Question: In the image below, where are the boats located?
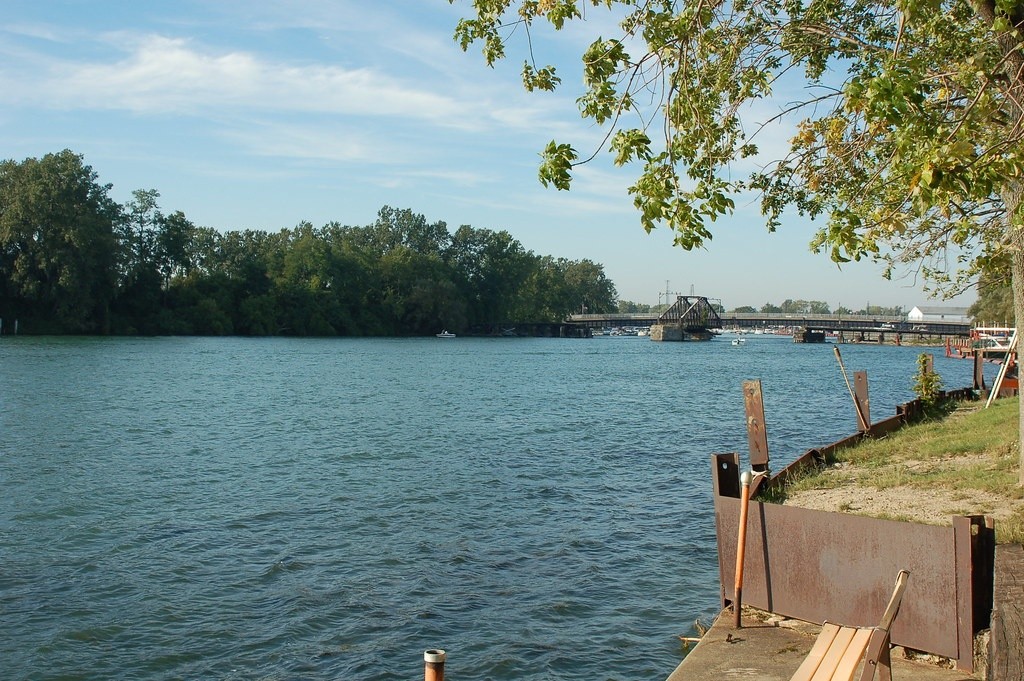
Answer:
[591,324,651,337]
[436,330,456,338]
[731,339,746,347]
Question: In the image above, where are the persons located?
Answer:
[1005,361,1019,380]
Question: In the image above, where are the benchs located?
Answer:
[789,570,910,681]
